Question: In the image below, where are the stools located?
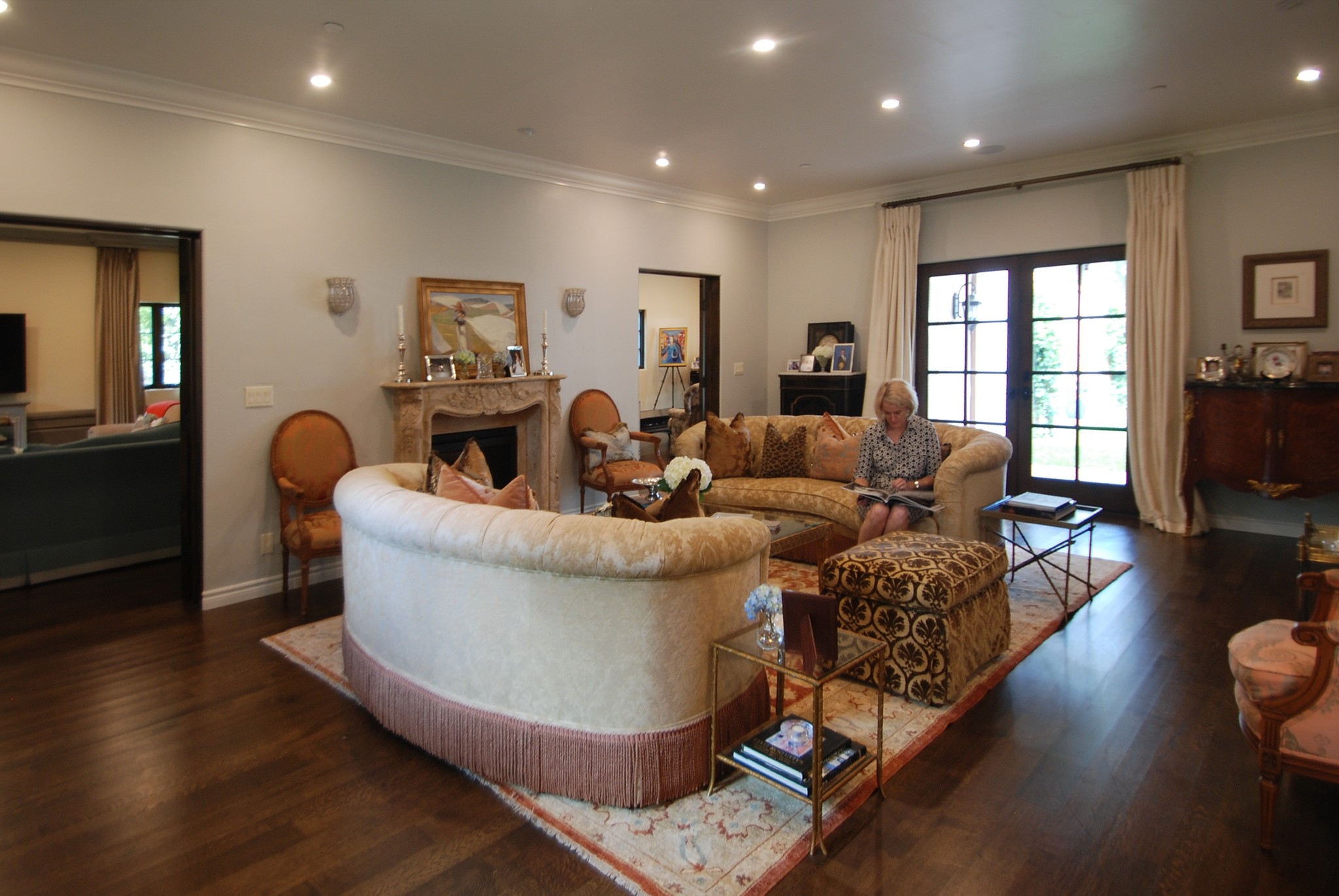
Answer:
[818,529,1012,709]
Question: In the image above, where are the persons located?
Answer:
[514,351,524,374]
[437,363,444,372]
[853,379,941,545]
[793,362,798,370]
[838,349,847,370]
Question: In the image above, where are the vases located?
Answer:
[494,362,507,377]
[457,363,470,380]
[755,609,783,652]
[816,354,829,373]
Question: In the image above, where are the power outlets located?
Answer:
[261,532,275,556]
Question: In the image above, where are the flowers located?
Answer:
[654,455,713,503]
[744,582,795,645]
[811,344,834,368]
[451,350,476,365]
[490,350,513,368]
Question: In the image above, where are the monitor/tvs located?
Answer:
[0,313,26,393]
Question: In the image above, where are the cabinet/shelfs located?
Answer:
[689,368,700,387]
[0,392,32,448]
[776,369,867,418]
[1177,375,1339,538]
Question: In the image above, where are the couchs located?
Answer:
[667,410,1015,566]
[331,436,773,813]
[0,399,183,592]
[666,382,701,455]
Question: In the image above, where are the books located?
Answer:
[733,714,867,797]
[839,482,945,512]
[999,491,1076,521]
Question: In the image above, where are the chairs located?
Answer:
[1225,565,1339,858]
[270,409,361,617]
[568,387,666,516]
[1294,509,1339,621]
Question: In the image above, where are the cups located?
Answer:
[477,355,494,378]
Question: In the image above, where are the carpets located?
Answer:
[259,538,1136,896]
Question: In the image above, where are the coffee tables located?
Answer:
[596,492,833,595]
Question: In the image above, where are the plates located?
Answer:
[1259,343,1298,380]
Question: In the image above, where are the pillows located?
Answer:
[820,411,852,440]
[754,422,811,479]
[434,463,541,512]
[581,423,638,476]
[422,437,495,496]
[610,467,705,525]
[702,412,756,481]
[806,422,864,483]
[132,414,167,432]
[940,441,953,463]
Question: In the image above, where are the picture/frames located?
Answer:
[424,354,457,381]
[1306,354,1339,382]
[780,588,839,672]
[787,358,801,372]
[658,327,688,367]
[417,276,530,382]
[1241,248,1331,331]
[1250,340,1310,380]
[830,342,856,373]
[505,345,528,378]
[798,353,816,373]
[1200,354,1225,381]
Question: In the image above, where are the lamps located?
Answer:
[953,281,983,334]
[566,288,587,317]
[326,277,355,314]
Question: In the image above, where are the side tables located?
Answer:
[977,493,1104,625]
[703,609,888,859]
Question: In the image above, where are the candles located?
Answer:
[396,305,405,335]
[542,308,547,334]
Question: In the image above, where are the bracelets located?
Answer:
[914,480,919,489]
